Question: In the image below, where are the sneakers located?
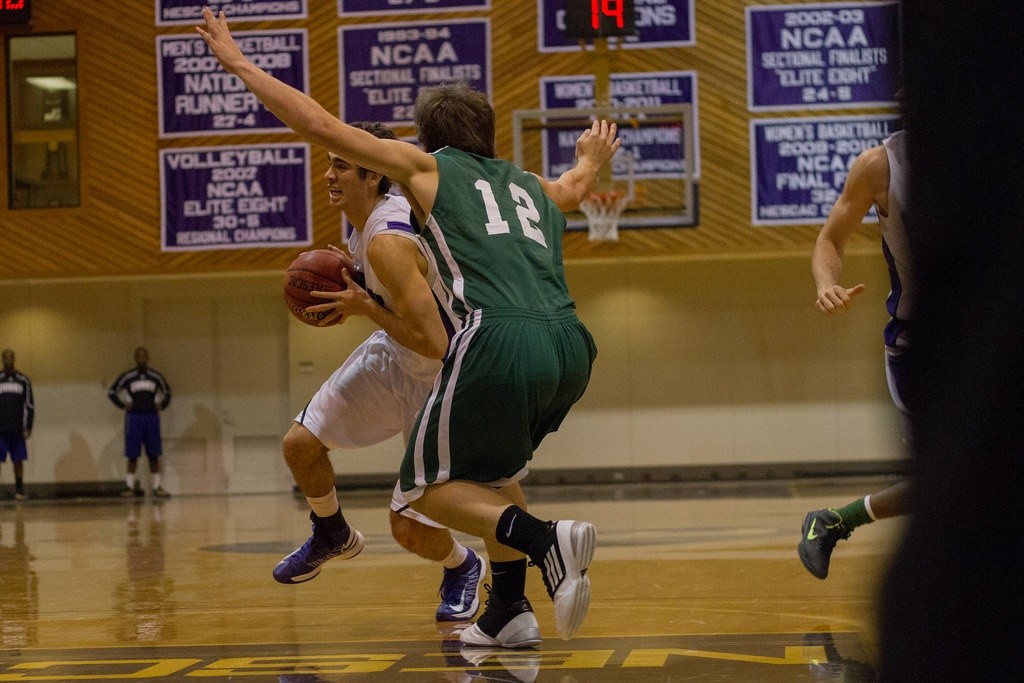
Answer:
[528,520,597,641]
[435,547,487,622]
[460,583,542,648]
[271,524,365,585]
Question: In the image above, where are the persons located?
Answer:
[107,346,172,500]
[193,6,622,649]
[798,81,914,581]
[0,348,35,502]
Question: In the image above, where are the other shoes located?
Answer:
[798,508,852,579]
[153,486,172,499]
[14,493,30,500]
[118,487,137,499]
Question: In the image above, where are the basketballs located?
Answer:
[283,248,357,328]
